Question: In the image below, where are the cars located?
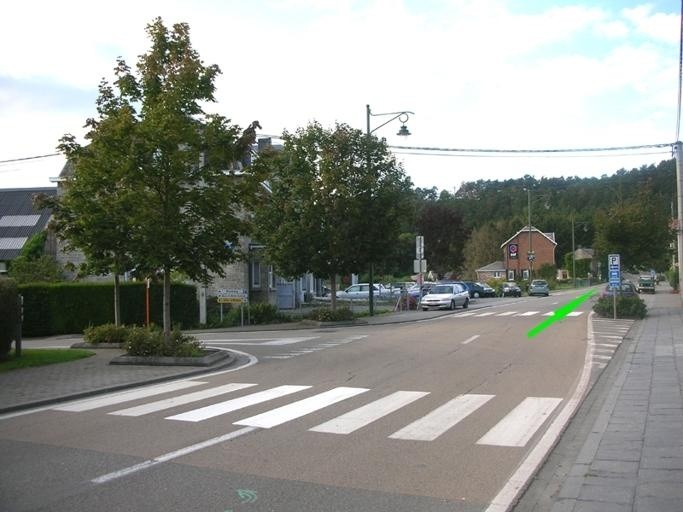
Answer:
[502,282,522,298]
[638,275,656,294]
[602,280,638,298]
[326,280,496,311]
[528,279,549,297]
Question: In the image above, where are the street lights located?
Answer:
[366,104,415,317]
